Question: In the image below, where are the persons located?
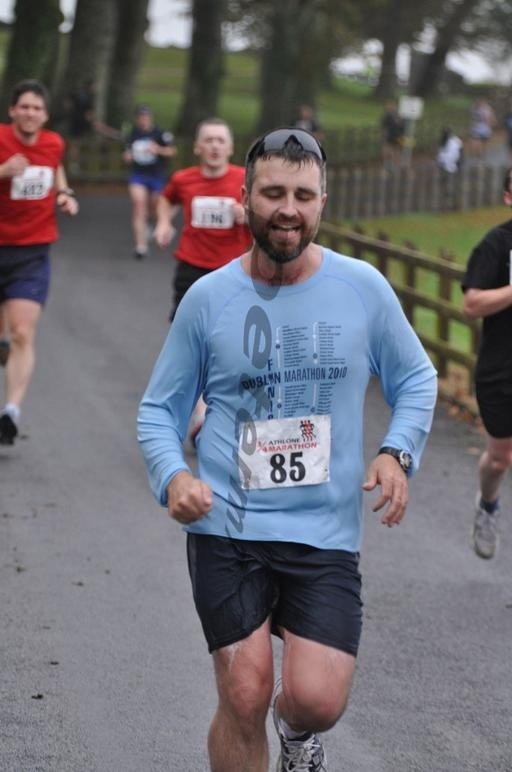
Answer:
[139,126,442,772]
[436,122,465,175]
[294,100,326,144]
[457,160,512,563]
[380,97,409,170]
[151,114,255,451]
[469,94,497,153]
[116,104,176,261]
[0,76,80,447]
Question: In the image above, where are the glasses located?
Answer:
[247,130,324,166]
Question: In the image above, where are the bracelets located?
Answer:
[55,186,75,195]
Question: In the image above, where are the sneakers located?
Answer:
[134,249,146,258]
[2,415,13,448]
[474,493,499,556]
[271,677,325,771]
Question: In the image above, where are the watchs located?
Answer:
[375,442,417,481]
